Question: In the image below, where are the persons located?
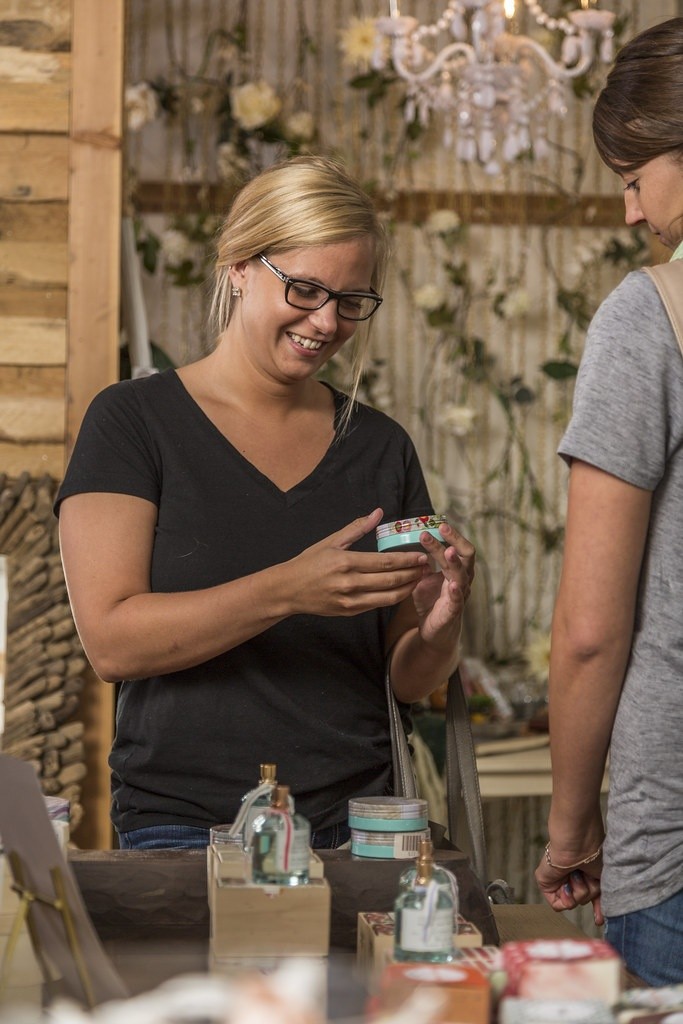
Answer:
[54,156,474,849]
[535,18,683,987]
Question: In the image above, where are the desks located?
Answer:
[70,850,502,955]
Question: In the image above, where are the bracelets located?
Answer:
[545,841,603,870]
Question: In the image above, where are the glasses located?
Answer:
[256,253,383,321]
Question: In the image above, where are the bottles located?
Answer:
[395,835,460,963]
[242,761,312,884]
